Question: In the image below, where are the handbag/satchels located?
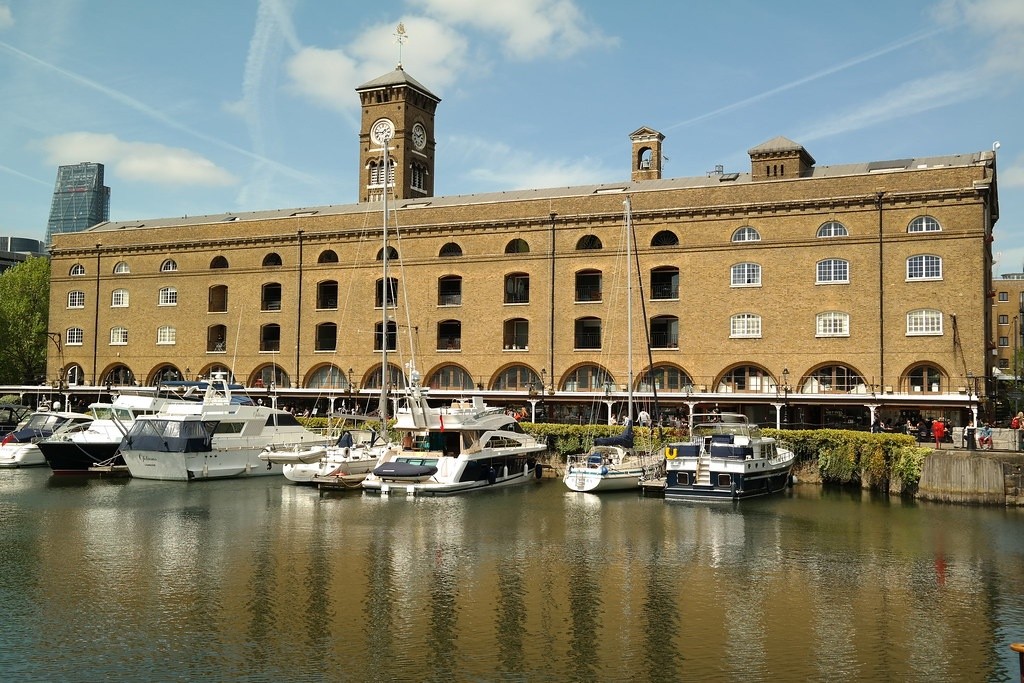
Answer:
[515,414,521,420]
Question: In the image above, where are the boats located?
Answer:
[0,304,340,483]
[664,412,795,504]
[257,446,327,465]
[361,360,547,497]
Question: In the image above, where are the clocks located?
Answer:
[371,118,395,145]
[412,123,426,151]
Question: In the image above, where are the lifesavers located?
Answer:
[666,448,677,459]
[343,447,350,457]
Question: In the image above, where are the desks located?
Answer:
[296,414,304,416]
[908,429,919,436]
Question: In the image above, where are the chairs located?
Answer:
[980,433,993,449]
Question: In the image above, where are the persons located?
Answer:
[978,422,992,450]
[964,421,973,440]
[895,418,903,426]
[885,418,893,425]
[508,407,527,422]
[873,419,886,433]
[623,415,628,426]
[636,408,650,426]
[933,417,952,448]
[291,403,296,415]
[658,415,663,425]
[1014,412,1024,429]
[918,421,926,432]
[303,407,311,417]
[610,414,615,425]
[401,432,414,450]
[283,403,289,411]
[904,420,912,429]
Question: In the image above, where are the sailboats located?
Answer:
[256,135,397,487]
[562,196,667,492]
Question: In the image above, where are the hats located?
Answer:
[984,423,990,425]
